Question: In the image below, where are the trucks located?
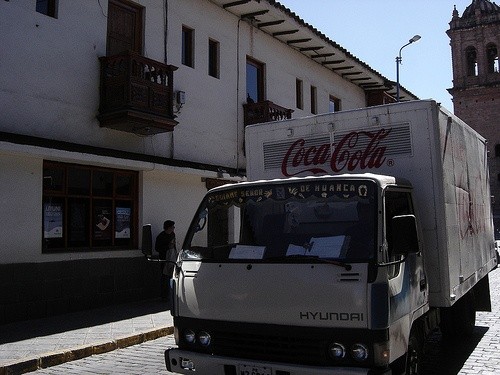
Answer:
[140,96,499,375]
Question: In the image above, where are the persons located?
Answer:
[348,202,372,256]
[154,220,177,311]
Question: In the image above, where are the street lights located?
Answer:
[395,34,422,102]
